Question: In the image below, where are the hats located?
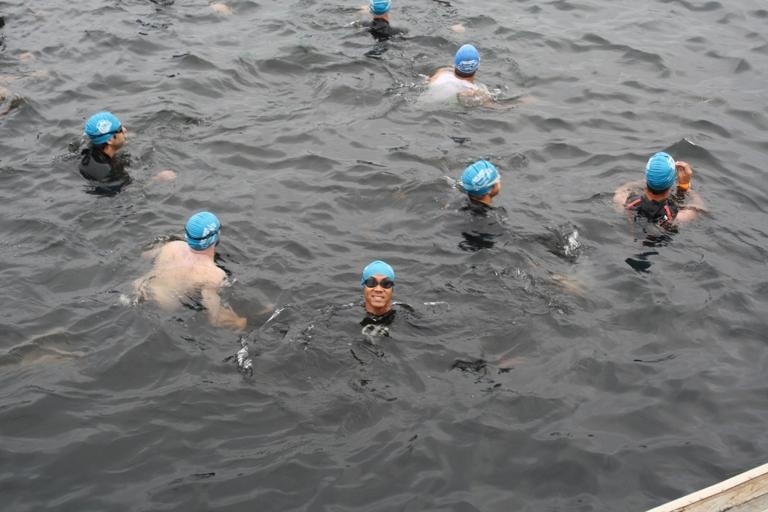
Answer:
[454,44,480,74]
[183,212,220,251]
[460,160,501,196]
[84,112,122,145]
[360,260,395,290]
[644,152,676,190]
[369,0,392,15]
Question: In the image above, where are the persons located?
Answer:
[413,43,538,112]
[132,209,277,331]
[81,110,177,197]
[337,258,416,340]
[349,1,467,45]
[608,147,710,229]
[450,156,508,239]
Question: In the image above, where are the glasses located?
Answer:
[365,277,394,289]
[109,125,122,135]
